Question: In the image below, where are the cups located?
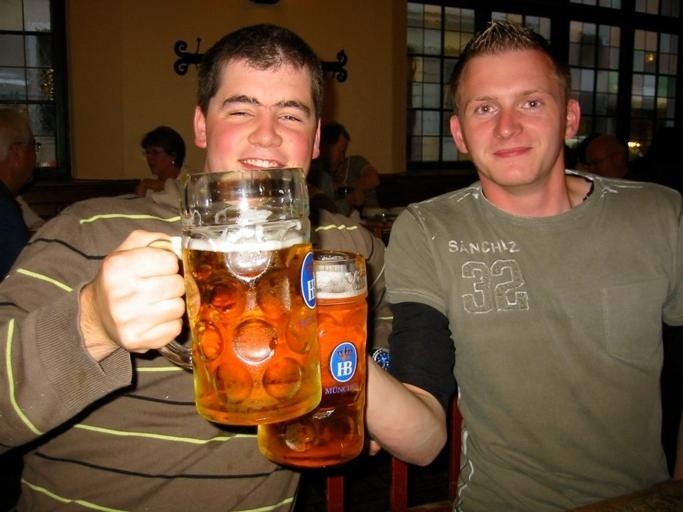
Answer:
[140,164,324,427]
[255,248,372,472]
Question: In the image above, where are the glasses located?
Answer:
[7,141,42,152]
[142,151,164,159]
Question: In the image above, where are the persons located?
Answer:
[305,121,349,226]
[0,22,395,509]
[136,124,198,195]
[580,132,633,180]
[364,12,681,512]
[0,102,60,279]
[333,151,382,217]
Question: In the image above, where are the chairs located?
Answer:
[327,391,462,512]
